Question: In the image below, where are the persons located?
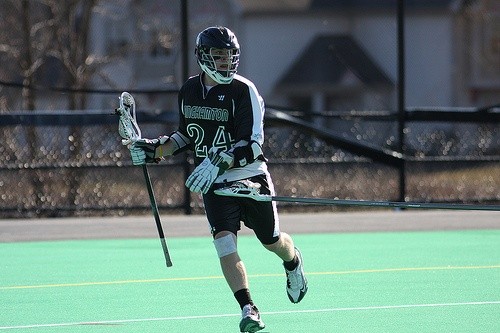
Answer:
[122,27,309,333]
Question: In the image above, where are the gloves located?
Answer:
[185,147,234,194]
[130,139,162,165]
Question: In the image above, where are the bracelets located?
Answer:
[159,146,162,158]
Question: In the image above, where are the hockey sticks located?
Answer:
[213,179,500,211]
[117,91,172,268]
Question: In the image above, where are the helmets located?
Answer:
[195,26,240,84]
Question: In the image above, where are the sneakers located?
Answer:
[240,304,265,332]
[283,246,308,303]
